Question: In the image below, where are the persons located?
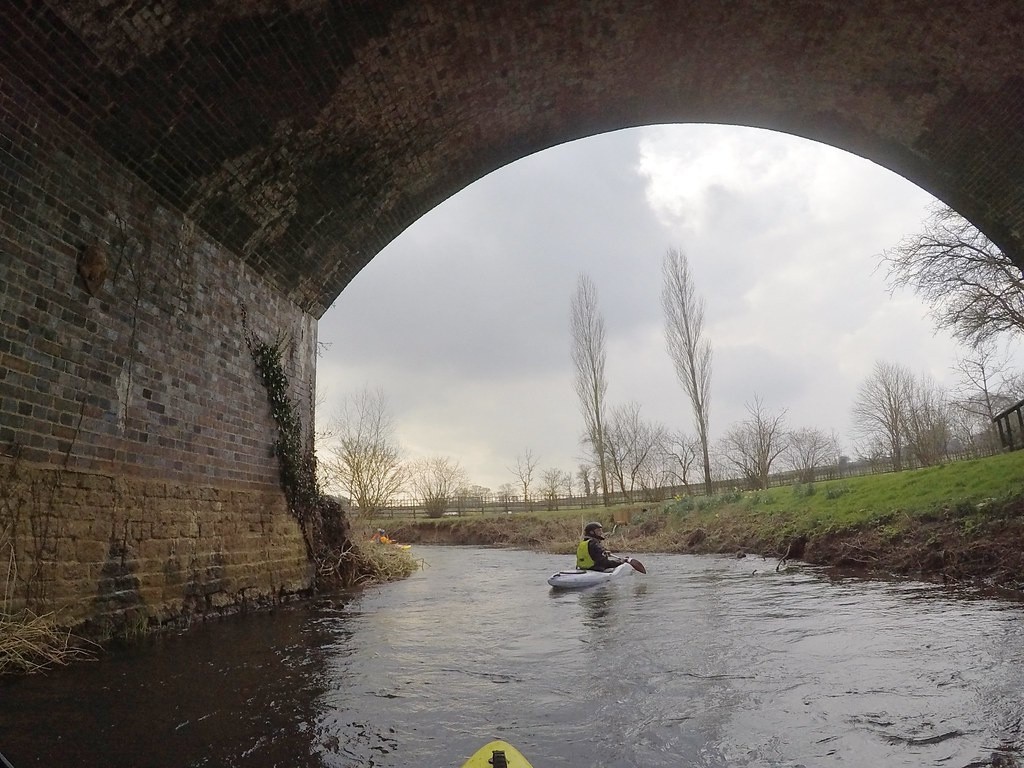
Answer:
[576,522,629,572]
[372,528,388,540]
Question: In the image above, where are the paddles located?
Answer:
[610,553,646,574]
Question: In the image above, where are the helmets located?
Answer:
[584,522,602,531]
[380,529,385,535]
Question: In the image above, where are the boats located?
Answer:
[369,540,412,550]
[547,555,635,589]
